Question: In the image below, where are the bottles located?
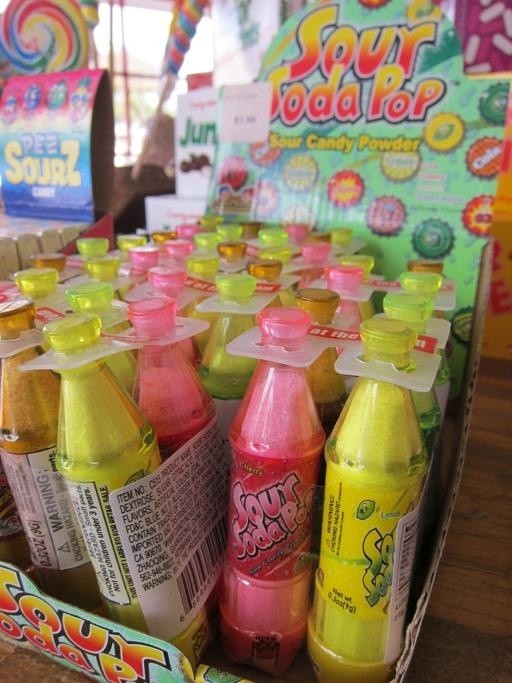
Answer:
[1,216,458,683]
